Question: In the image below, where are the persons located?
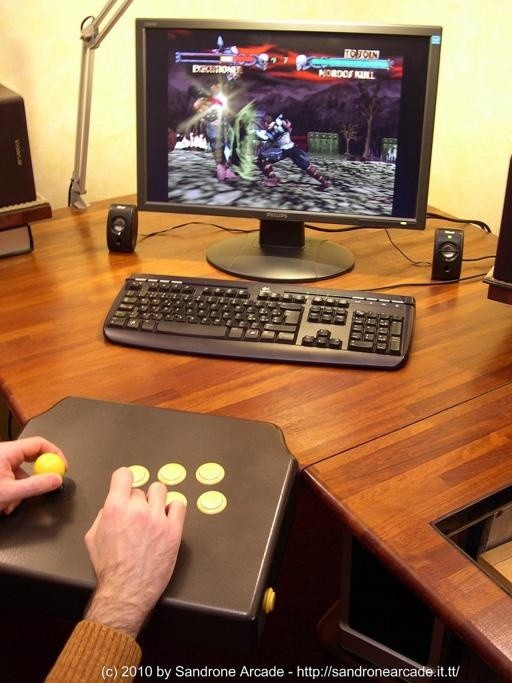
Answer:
[193,85,238,177]
[248,112,332,192]
[1,437,186,682]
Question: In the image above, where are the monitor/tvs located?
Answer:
[136,18,442,230]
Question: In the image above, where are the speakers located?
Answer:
[432,230,465,282]
[107,204,137,252]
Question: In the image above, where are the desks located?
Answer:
[0,191,512,683]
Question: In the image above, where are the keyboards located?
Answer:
[102,271,416,371]
